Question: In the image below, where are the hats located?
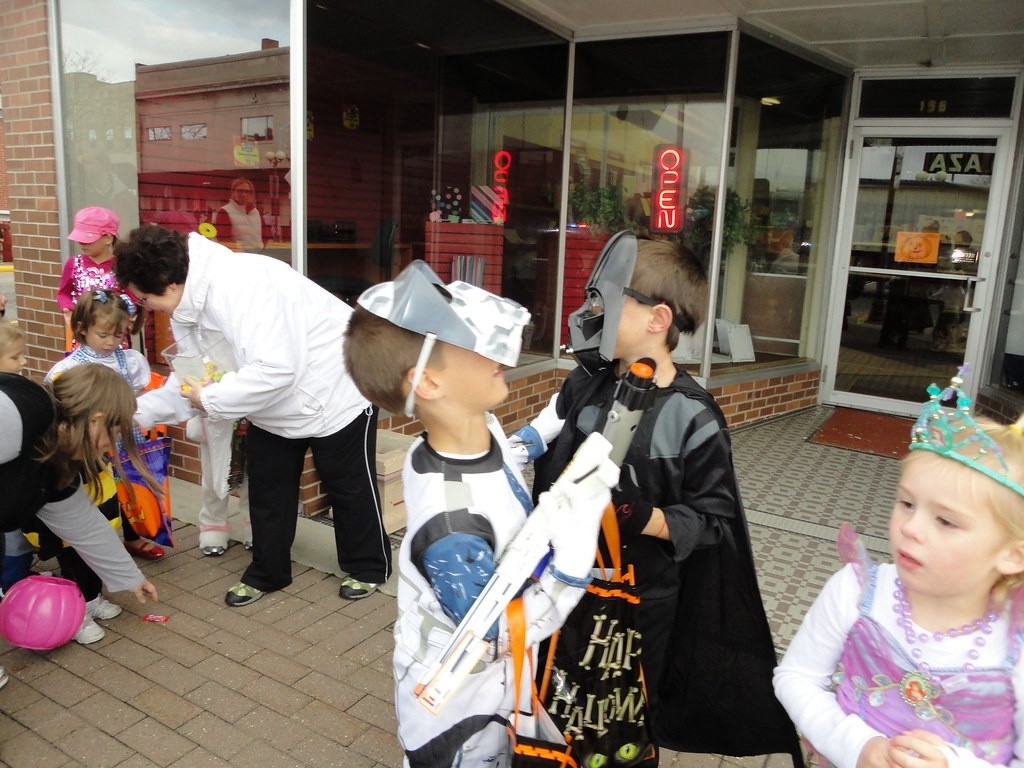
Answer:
[67,206,120,244]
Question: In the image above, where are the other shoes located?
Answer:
[924,337,949,353]
[0,571,58,604]
[876,330,909,351]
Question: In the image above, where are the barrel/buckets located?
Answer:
[161,329,239,392]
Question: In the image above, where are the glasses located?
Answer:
[233,188,252,194]
[133,292,149,308]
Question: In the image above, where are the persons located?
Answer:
[113,224,393,607]
[841,219,973,356]
[773,361,1024,767]
[0,322,41,597]
[44,289,166,559]
[526,229,802,768]
[0,364,159,688]
[215,177,265,253]
[44,448,125,644]
[56,206,145,352]
[189,410,254,556]
[343,258,615,768]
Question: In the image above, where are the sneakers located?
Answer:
[71,613,105,646]
[85,592,122,620]
[244,541,253,550]
[338,575,380,599]
[202,545,224,556]
[225,582,268,606]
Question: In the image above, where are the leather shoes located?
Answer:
[122,540,164,561]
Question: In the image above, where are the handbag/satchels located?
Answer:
[105,424,174,548]
[506,596,583,768]
[532,498,660,768]
